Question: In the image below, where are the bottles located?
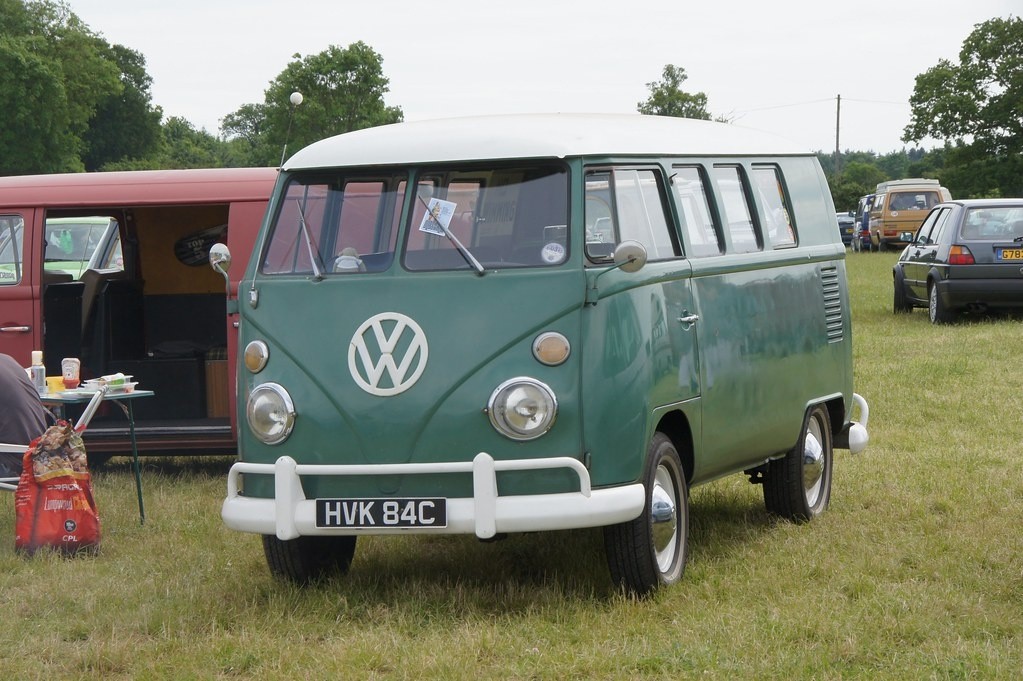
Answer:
[30,350,46,397]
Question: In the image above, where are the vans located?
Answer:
[864,178,954,252]
[849,194,876,252]
[0,171,484,473]
[207,112,870,603]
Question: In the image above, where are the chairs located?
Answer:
[42,273,86,368]
[77,267,128,346]
[964,225,979,238]
[1011,220,1023,238]
[0,384,109,554]
[914,201,925,210]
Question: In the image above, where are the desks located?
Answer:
[40,390,155,526]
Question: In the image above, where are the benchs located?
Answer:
[204,339,230,418]
[509,243,614,265]
[105,280,204,420]
[320,245,499,273]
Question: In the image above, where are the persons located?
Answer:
[0,353,48,486]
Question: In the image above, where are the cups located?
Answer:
[47,377,65,394]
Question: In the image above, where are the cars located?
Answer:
[836,211,857,245]
[892,198,1023,326]
